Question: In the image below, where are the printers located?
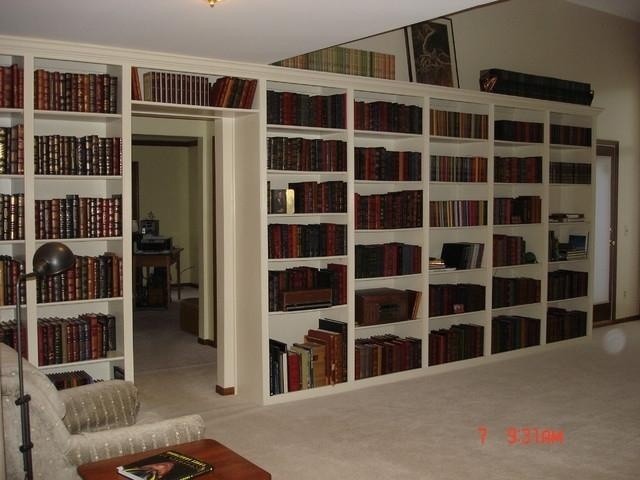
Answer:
[140,235,173,252]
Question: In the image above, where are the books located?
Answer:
[117,450,212,480]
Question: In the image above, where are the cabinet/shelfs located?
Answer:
[427,92,491,368]
[546,107,591,350]
[127,61,261,115]
[491,101,544,363]
[349,79,426,389]
[0,51,134,390]
[236,71,352,408]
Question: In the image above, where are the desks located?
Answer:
[78,436,271,479]
[138,248,183,309]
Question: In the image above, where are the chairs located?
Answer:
[0,335,207,480]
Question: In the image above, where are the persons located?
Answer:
[0,58,589,396]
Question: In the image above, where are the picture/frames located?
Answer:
[405,18,461,87]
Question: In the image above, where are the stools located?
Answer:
[180,297,198,336]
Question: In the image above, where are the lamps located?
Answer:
[15,242,76,480]
